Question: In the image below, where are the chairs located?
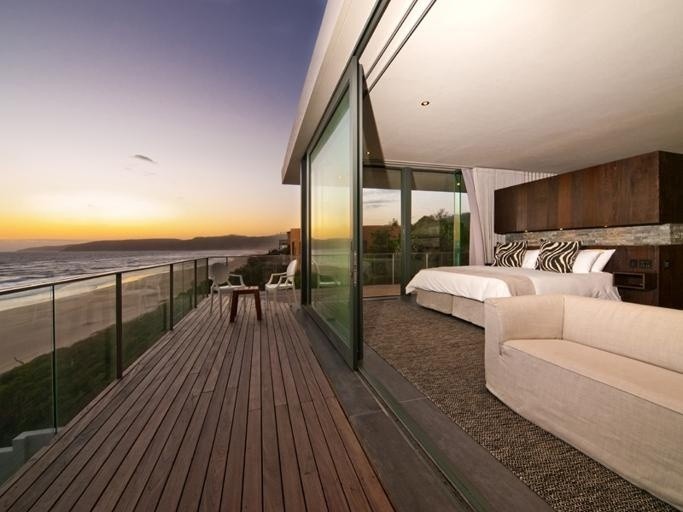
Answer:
[311,260,341,309]
[263,259,297,316]
[207,260,246,317]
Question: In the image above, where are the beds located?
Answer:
[404,245,623,330]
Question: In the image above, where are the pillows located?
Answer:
[521,250,536,267]
[584,248,616,272]
[572,250,604,273]
[492,240,528,268]
[532,239,581,273]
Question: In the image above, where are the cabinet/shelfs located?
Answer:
[526,174,560,231]
[494,176,527,233]
[606,151,659,228]
[560,162,606,228]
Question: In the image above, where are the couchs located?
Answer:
[483,292,682,509]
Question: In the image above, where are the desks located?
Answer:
[229,285,261,322]
